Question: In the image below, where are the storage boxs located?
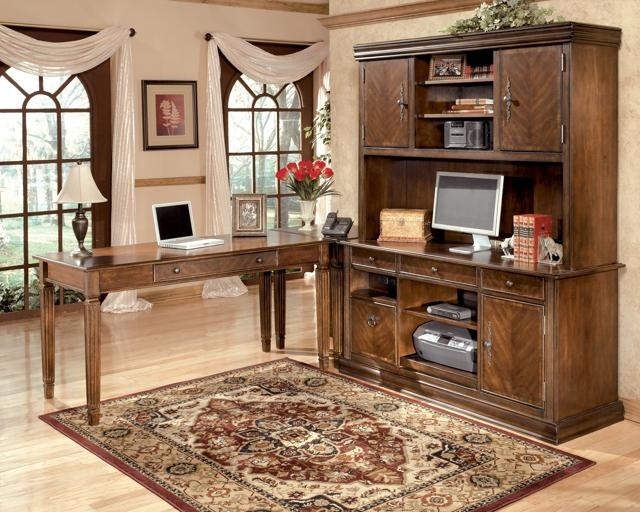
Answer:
[378,209,432,244]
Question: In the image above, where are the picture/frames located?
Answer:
[232,194,267,239]
[141,80,199,151]
[428,54,465,80]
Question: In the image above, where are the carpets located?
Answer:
[39,357,596,512]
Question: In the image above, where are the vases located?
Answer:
[297,200,318,231]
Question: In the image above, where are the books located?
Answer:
[444,97,497,114]
[465,65,494,79]
[513,213,554,266]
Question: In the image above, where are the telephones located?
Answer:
[321,210,355,238]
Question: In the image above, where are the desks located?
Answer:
[31,230,333,426]
[269,224,359,368]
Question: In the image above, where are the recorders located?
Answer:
[444,117,491,150]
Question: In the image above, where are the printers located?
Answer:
[413,321,478,374]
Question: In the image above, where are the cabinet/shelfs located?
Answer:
[332,21,624,445]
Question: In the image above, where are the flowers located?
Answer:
[276,161,341,201]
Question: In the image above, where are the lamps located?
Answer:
[52,161,107,259]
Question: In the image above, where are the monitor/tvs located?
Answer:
[432,170,503,254]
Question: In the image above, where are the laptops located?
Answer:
[152,200,225,250]
